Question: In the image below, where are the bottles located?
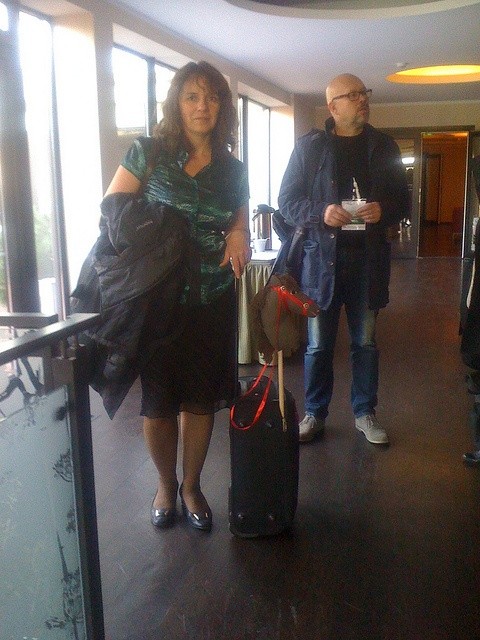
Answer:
[252,203,275,251]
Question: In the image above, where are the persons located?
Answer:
[277,73,409,443]
[102,60,252,529]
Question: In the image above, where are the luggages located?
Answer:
[229,349,300,540]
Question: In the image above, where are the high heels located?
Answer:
[151,479,179,527]
[179,483,215,531]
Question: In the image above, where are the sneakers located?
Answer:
[298,409,326,445]
[355,413,389,445]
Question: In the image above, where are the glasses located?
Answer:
[334,88,373,101]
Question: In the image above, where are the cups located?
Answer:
[253,239,267,253]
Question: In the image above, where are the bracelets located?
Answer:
[225,228,250,235]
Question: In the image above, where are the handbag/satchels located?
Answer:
[266,213,301,287]
